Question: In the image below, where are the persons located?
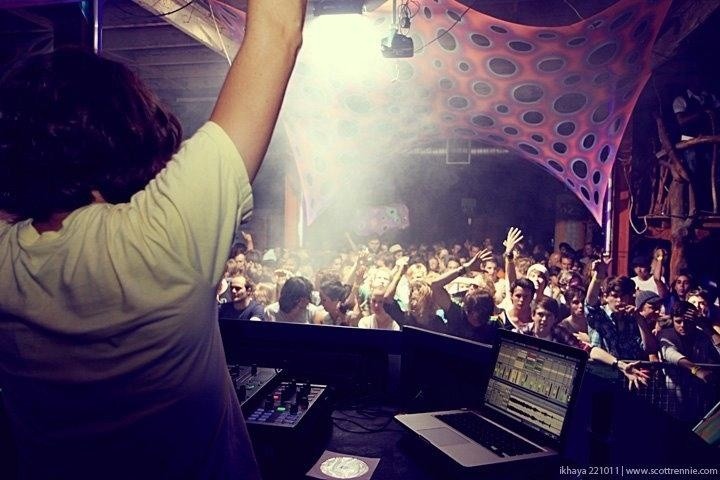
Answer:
[0,1,307,480]
[220,226,719,390]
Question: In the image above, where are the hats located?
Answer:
[389,244,402,253]
[526,263,549,278]
[632,256,650,266]
[634,291,662,309]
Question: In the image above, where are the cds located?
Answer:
[320,457,369,479]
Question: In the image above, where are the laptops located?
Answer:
[391,328,588,480]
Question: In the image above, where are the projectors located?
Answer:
[380,36,414,57]
